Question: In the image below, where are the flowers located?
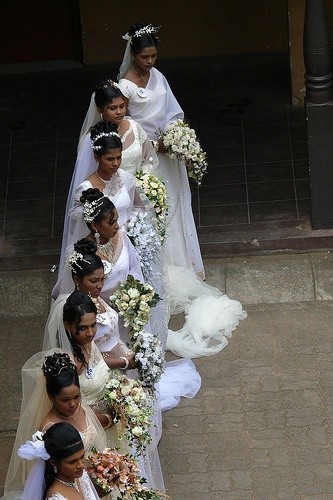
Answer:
[87,117,208,500]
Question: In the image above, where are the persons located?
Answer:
[41,290,118,498]
[17,420,101,500]
[39,352,123,500]
[116,19,205,282]
[61,79,168,413]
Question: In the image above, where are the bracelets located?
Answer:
[102,414,112,429]
[151,140,159,153]
[118,356,130,371]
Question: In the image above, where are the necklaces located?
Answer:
[95,169,110,184]
[51,405,81,423]
[77,343,92,357]
[87,234,116,263]
[54,477,80,493]
[116,120,121,131]
[132,64,148,84]
